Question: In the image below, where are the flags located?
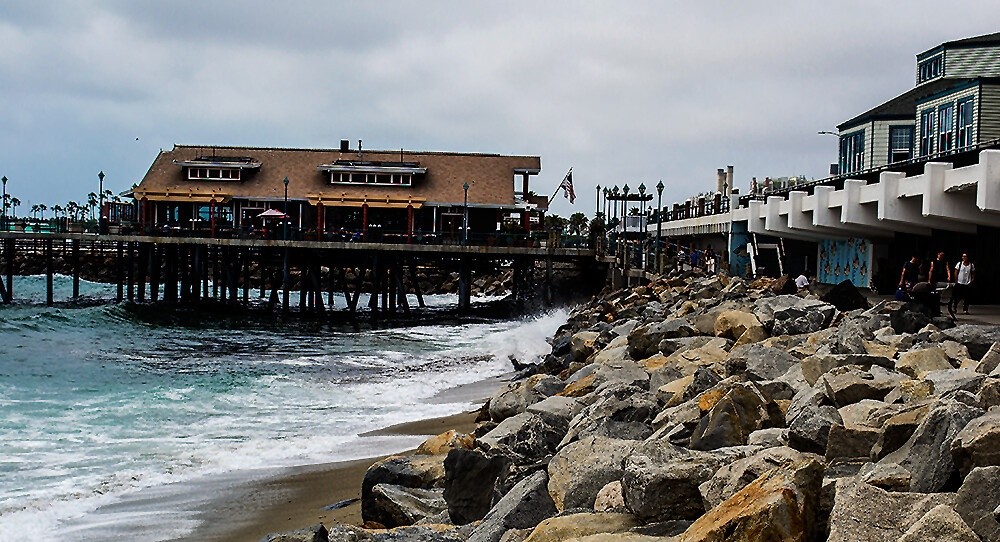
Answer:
[562,169,576,204]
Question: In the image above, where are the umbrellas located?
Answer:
[256,208,289,219]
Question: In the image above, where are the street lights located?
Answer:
[602,187,607,225]
[98,171,105,235]
[656,180,664,255]
[594,184,601,217]
[621,184,629,247]
[606,188,612,225]
[2,176,8,223]
[613,184,619,220]
[637,183,647,254]
[462,181,469,249]
[282,177,289,240]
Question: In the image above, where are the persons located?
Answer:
[952,252,977,314]
[794,270,810,289]
[898,253,921,297]
[645,233,717,274]
[927,249,951,284]
